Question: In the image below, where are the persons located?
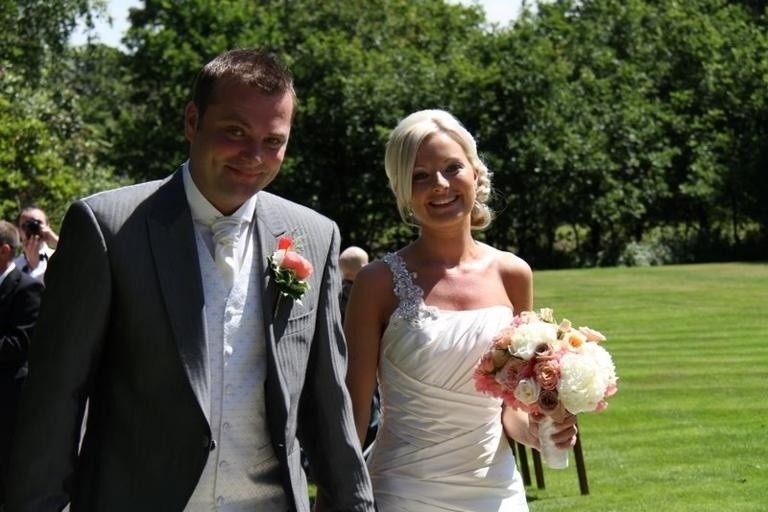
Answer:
[1,49,374,512]
[14,207,59,279]
[342,108,578,512]
[340,245,389,330]
[0,221,46,392]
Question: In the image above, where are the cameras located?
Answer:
[18,219,43,240]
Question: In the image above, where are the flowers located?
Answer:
[471,307,620,426]
[267,226,316,307]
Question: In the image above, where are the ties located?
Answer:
[210,218,241,289]
